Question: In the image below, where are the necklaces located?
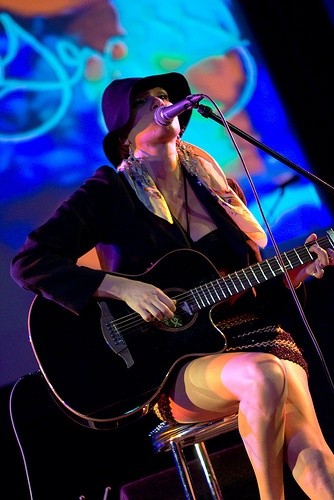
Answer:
[158,167,191,242]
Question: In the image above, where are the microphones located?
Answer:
[153,93,206,127]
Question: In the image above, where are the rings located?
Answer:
[312,271,316,277]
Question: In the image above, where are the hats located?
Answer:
[101,72,192,169]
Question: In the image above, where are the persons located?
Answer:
[8,73,334,500]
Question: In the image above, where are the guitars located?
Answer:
[27,226,334,421]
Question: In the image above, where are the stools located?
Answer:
[148,409,241,499]
